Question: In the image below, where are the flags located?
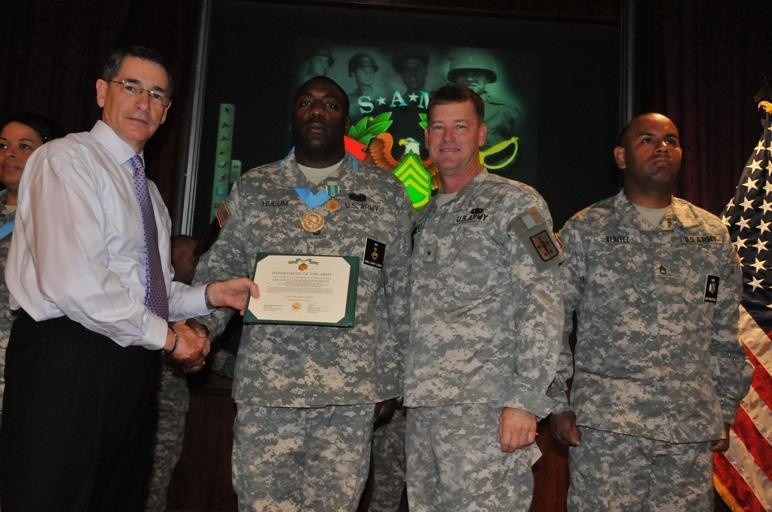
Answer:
[709,115,771,512]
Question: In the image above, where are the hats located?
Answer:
[392,45,429,74]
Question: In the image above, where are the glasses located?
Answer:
[105,79,171,108]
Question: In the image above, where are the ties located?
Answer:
[130,155,168,322]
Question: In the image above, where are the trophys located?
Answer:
[301,211,325,232]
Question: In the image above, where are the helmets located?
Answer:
[446,47,498,83]
[349,53,377,77]
[313,46,333,66]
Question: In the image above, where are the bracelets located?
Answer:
[166,323,178,355]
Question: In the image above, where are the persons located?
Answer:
[1,117,47,407]
[145,234,236,511]
[301,34,528,174]
[548,112,755,511]
[400,84,566,511]
[370,399,412,511]
[2,40,260,510]
[175,77,414,511]
